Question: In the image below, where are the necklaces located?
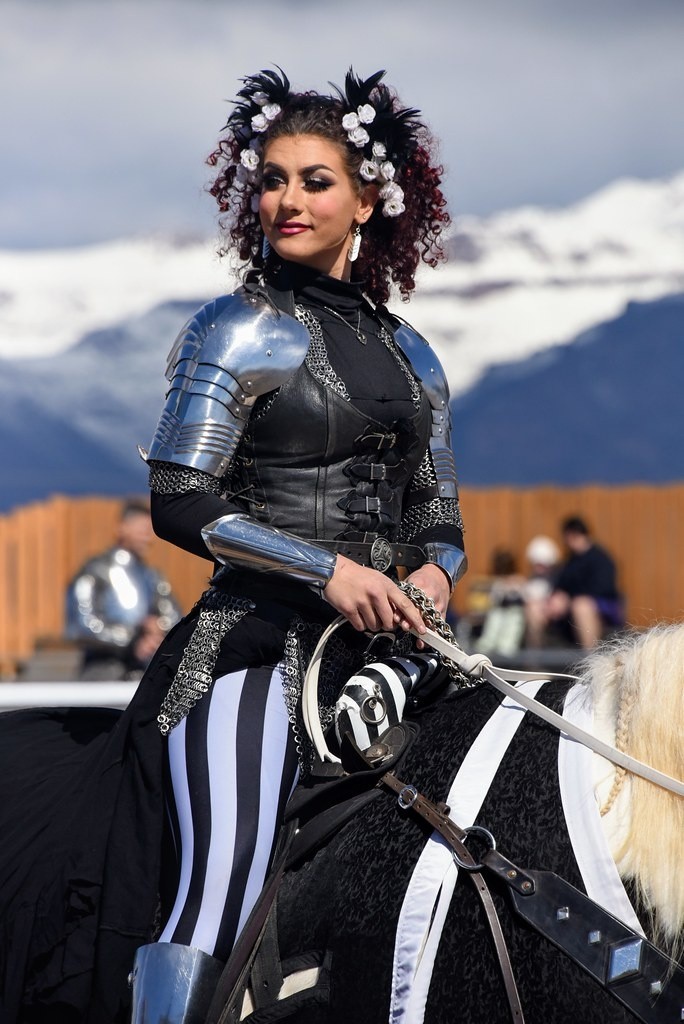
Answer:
[321,306,367,346]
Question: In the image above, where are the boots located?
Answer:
[127,942,225,1023]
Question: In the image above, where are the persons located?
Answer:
[0,64,469,1024]
[465,517,627,657]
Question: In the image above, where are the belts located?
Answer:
[301,538,429,573]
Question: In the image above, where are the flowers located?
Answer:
[379,182,404,202]
[348,126,370,148]
[249,91,269,106]
[251,113,270,132]
[371,140,387,164]
[239,148,260,171]
[262,103,281,121]
[377,161,395,184]
[342,112,360,132]
[250,193,260,212]
[381,199,406,218]
[357,104,376,124]
[359,160,381,181]
[236,163,253,185]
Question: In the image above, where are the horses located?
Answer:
[0,625,684,1024]
